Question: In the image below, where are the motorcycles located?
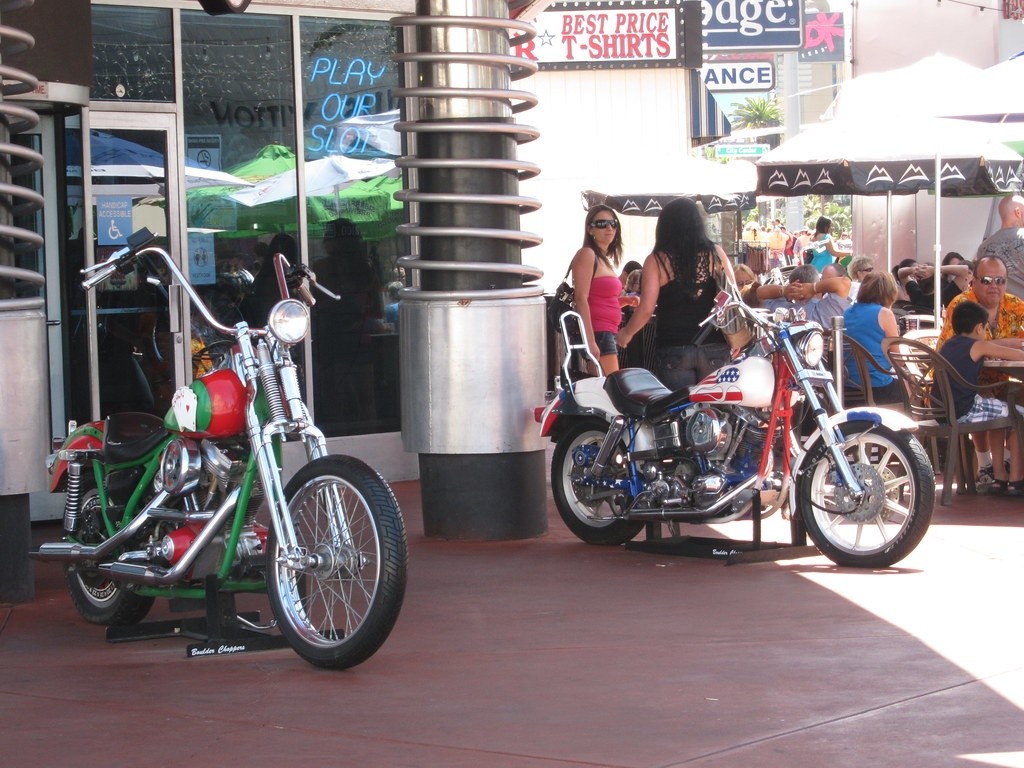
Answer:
[26,226,410,670]
[535,290,936,569]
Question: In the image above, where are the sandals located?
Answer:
[1004,481,1024,496]
[990,479,1008,495]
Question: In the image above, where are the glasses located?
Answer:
[736,280,753,288]
[856,266,873,273]
[588,218,618,229]
[973,273,1008,285]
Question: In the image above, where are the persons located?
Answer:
[619,217,974,473]
[181,218,406,381]
[931,255,1024,494]
[573,205,640,377]
[619,198,753,395]
[931,302,1024,494]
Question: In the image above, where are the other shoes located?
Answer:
[974,459,1010,496]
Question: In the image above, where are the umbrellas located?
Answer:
[64,109,404,281]
[580,55,1024,339]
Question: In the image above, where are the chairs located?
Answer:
[823,315,1024,507]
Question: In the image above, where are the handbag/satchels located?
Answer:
[710,242,752,349]
[547,245,598,337]
